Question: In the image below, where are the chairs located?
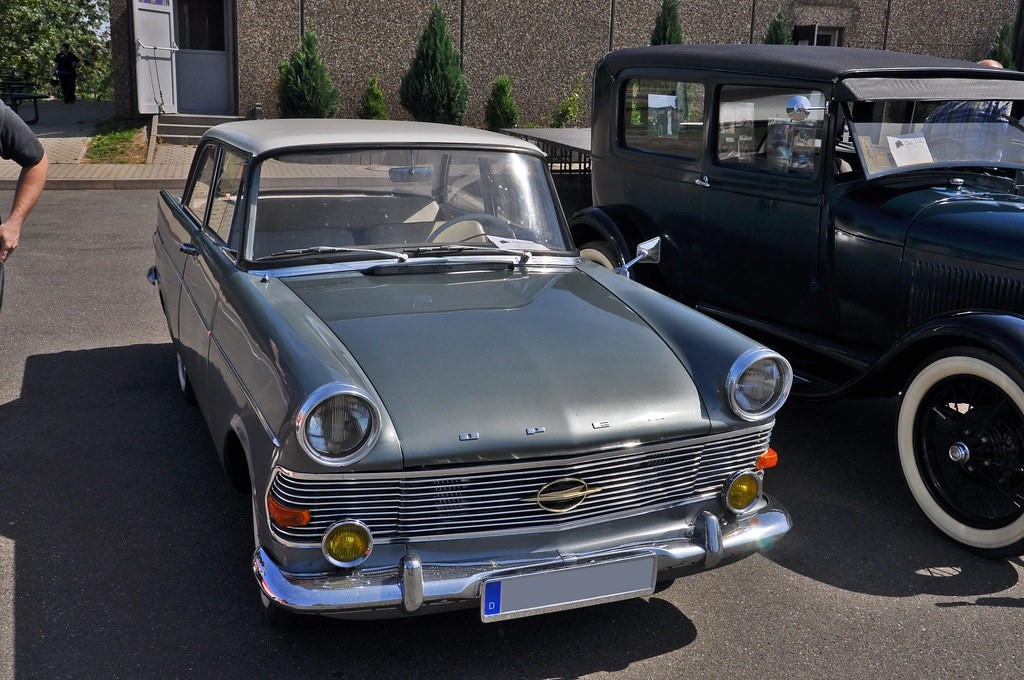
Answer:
[255,228,356,260]
[366,220,487,245]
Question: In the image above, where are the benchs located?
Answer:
[0,85,50,99]
[259,194,440,247]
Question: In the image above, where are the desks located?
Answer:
[0,80,39,124]
[501,128,591,210]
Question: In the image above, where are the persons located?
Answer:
[50,42,84,107]
[911,60,1024,162]
[0,99,48,317]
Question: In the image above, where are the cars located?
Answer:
[147,117,797,625]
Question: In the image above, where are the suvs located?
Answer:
[559,42,1024,558]
